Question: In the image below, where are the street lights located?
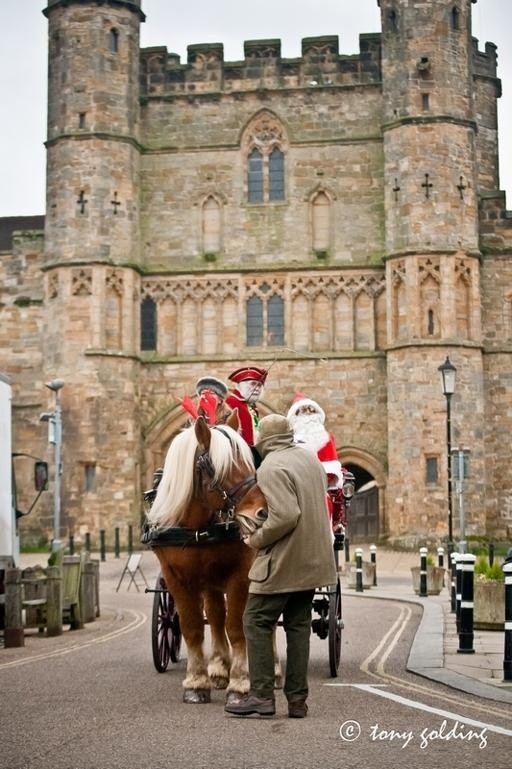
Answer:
[438,353,459,615]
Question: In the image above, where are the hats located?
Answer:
[228,366,268,384]
[195,375,227,401]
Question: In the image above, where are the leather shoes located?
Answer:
[224,696,276,716]
[287,702,309,718]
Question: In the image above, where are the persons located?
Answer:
[195,366,342,718]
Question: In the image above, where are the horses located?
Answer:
[142,407,270,708]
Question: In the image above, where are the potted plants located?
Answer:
[470,551,506,631]
[346,557,376,589]
[410,554,446,595]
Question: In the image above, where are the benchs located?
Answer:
[21,551,84,632]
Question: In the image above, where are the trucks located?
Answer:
[0,372,51,635]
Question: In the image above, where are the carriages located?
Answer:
[138,404,349,710]
